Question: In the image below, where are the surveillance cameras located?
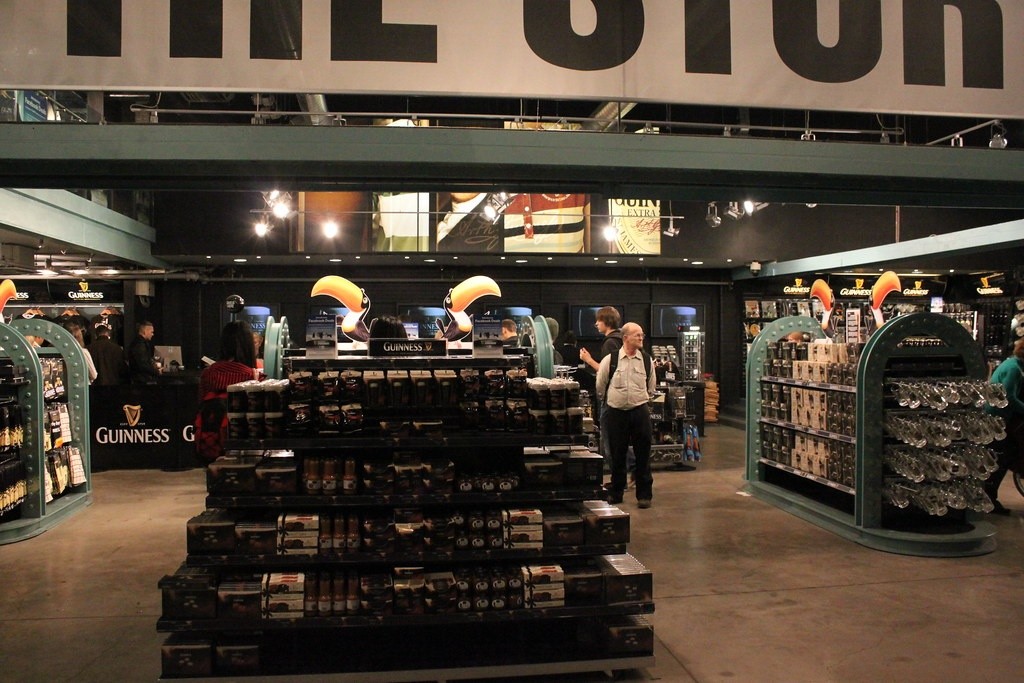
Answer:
[750,261,761,276]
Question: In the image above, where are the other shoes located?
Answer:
[603,490,625,506]
[636,497,652,510]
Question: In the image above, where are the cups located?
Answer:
[882,477,994,516]
[882,446,999,484]
[890,381,1008,411]
[882,413,1007,449]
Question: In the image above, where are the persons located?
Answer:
[788,332,803,343]
[982,337,1024,514]
[580,305,636,493]
[596,322,656,508]
[11,315,164,385]
[435,192,500,251]
[195,320,263,462]
[502,317,592,392]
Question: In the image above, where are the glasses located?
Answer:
[631,333,646,340]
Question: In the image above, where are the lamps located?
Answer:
[724,201,745,221]
[252,214,275,237]
[988,122,1008,149]
[745,200,770,216]
[663,218,680,238]
[704,201,722,229]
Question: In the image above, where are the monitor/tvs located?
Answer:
[219,302,281,338]
[569,304,625,341]
[397,303,452,339]
[307,302,350,319]
[484,303,541,323]
[154,346,183,370]
[651,303,706,337]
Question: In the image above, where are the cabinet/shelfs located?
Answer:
[600,386,685,469]
[0,318,92,542]
[154,353,656,683]
[680,331,701,382]
[739,295,1014,556]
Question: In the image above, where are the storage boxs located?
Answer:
[157,366,655,678]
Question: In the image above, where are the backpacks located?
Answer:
[192,384,235,465]
